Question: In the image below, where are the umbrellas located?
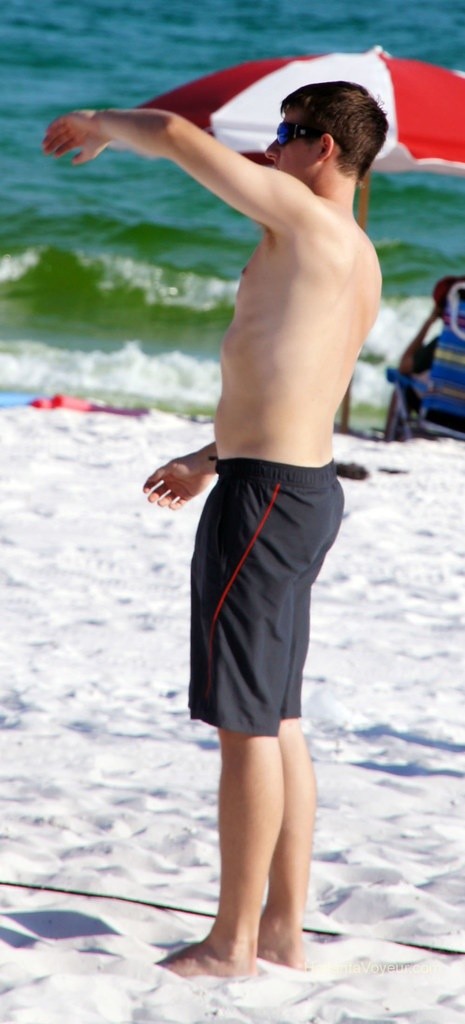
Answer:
[119,44,465,172]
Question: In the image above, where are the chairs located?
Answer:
[386,282,464,441]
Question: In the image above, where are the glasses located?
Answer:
[277,121,322,145]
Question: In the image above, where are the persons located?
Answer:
[388,274,463,442]
[36,78,393,982]
